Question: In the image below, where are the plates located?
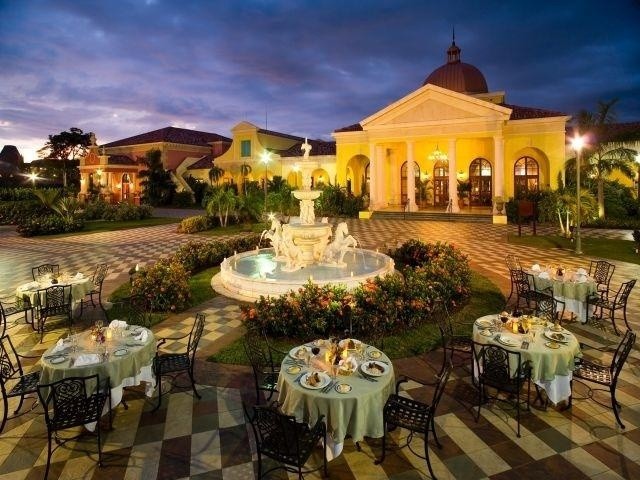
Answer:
[476,314,571,352]
[283,337,391,394]
[19,272,82,291]
[47,319,145,369]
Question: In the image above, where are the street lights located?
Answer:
[261,152,274,218]
[570,137,587,255]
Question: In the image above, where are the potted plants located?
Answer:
[416,177,435,207]
[456,179,473,208]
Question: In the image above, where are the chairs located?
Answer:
[242,400,329,480]
[342,305,353,337]
[504,254,638,336]
[126,294,153,330]
[2,304,44,433]
[431,298,473,377]
[152,312,206,410]
[561,330,637,430]
[242,327,289,405]
[374,359,454,480]
[471,341,531,438]
[15,261,112,343]
[35,374,115,476]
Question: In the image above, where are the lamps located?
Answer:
[427,141,448,162]
